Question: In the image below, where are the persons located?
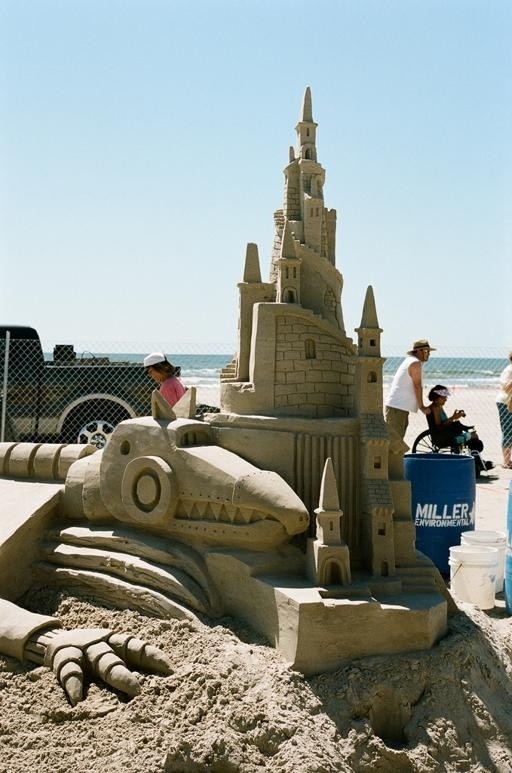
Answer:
[144,352,186,407]
[427,383,495,471]
[385,338,433,438]
[496,350,511,467]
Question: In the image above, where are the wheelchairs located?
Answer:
[409,405,497,479]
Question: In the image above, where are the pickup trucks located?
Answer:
[1,322,186,453]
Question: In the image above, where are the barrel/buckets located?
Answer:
[458,530,507,594]
[504,480,512,617]
[446,545,499,610]
[403,453,476,583]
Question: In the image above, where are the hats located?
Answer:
[408,339,438,355]
[432,384,450,397]
[144,352,166,367]
